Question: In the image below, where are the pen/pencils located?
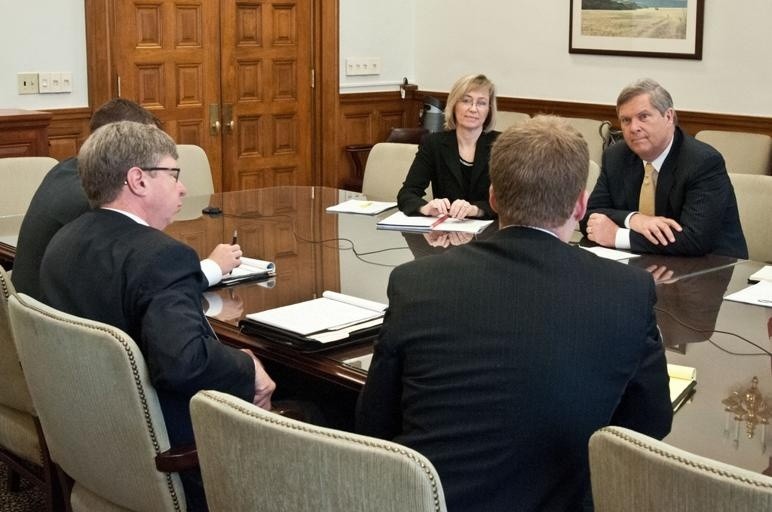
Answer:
[229,230,238,275]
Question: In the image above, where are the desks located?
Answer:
[343,144,374,193]
[0,186,772,512]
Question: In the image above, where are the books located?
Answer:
[375,211,448,231]
[326,200,397,215]
[585,245,642,261]
[748,265,772,282]
[245,289,390,338]
[665,361,698,411]
[219,255,276,285]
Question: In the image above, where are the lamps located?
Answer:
[721,376,772,455]
[387,78,445,143]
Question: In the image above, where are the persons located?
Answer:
[401,219,497,259]
[39,121,277,512]
[578,78,750,260]
[395,73,504,219]
[352,116,674,512]
[10,98,243,312]
[630,253,737,348]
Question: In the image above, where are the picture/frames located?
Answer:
[569,0,704,60]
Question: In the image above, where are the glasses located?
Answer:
[460,98,489,107]
[123,165,181,187]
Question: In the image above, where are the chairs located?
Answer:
[171,144,216,198]
[1,157,60,218]
[188,389,449,512]
[7,292,313,512]
[588,425,772,512]
[0,266,69,512]
[175,195,211,221]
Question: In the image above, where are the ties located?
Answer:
[639,163,657,218]
